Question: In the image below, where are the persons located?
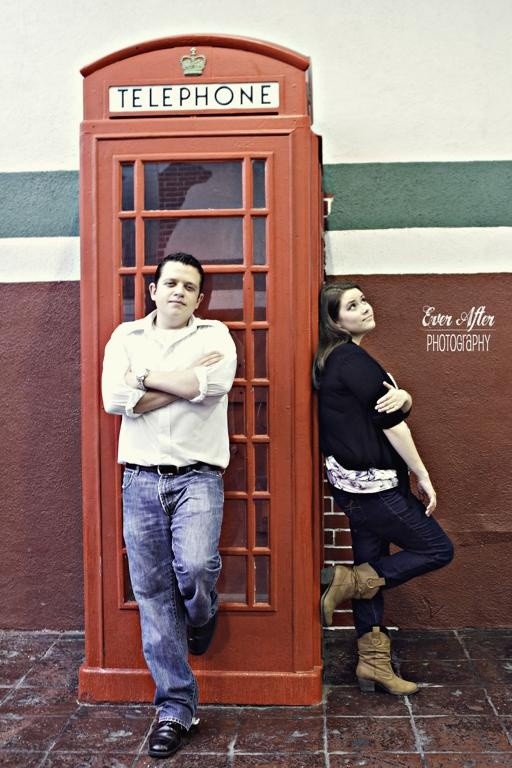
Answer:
[100,250,240,759]
[311,280,455,700]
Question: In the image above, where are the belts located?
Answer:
[126,461,206,476]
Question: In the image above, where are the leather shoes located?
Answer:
[187,614,216,657]
[149,721,197,757]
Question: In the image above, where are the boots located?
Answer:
[356,627,421,696]
[320,562,386,627]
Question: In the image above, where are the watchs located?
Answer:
[135,367,151,391]
